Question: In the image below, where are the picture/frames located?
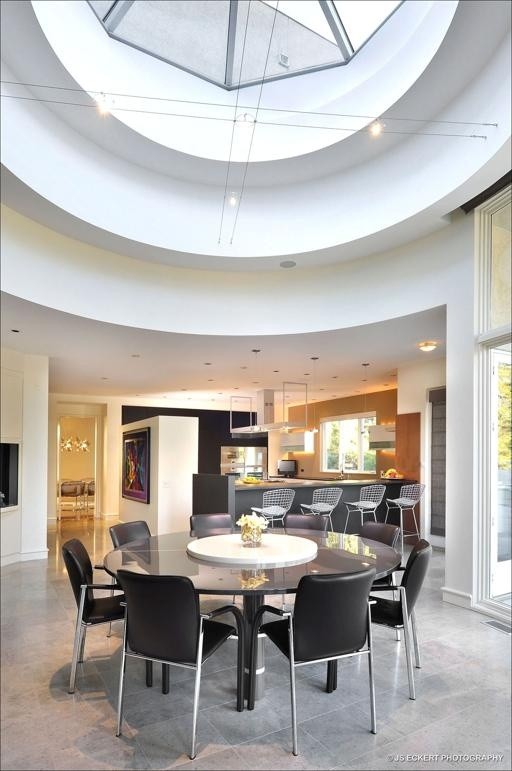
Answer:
[121,425,149,503]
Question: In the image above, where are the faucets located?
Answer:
[339,462,344,476]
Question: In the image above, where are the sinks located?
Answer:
[322,476,345,481]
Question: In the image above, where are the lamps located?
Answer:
[58,434,90,453]
[418,340,438,352]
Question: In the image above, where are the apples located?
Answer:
[389,472,396,478]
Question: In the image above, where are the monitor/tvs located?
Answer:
[278,460,296,477]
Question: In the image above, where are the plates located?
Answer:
[381,477,406,480]
[243,481,261,483]
